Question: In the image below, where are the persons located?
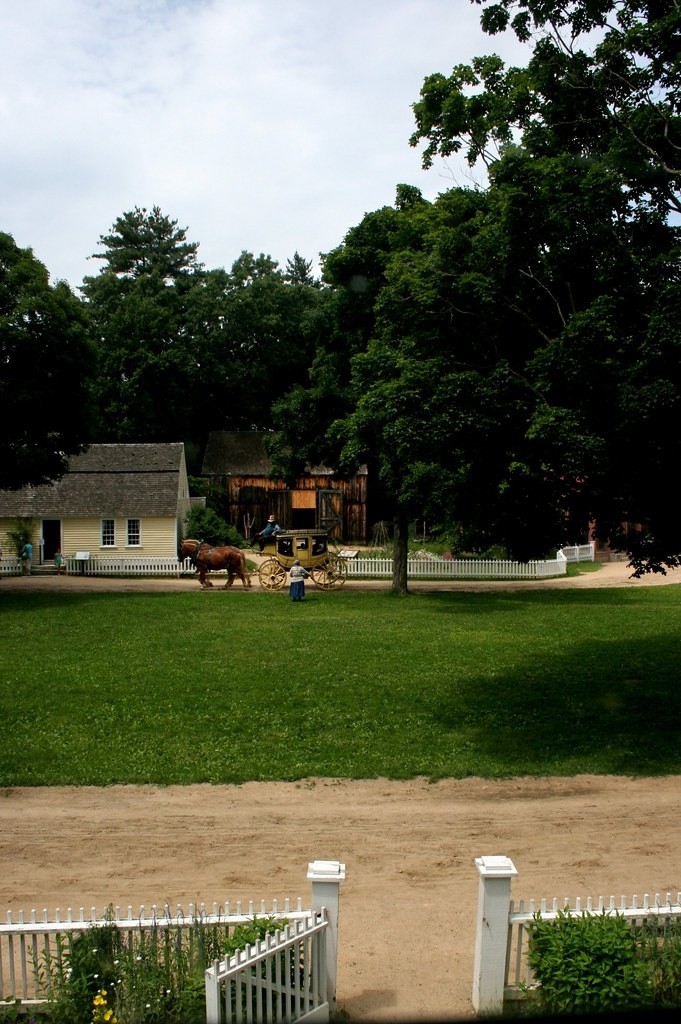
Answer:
[54,548,64,576]
[290,560,311,602]
[248,514,281,551]
[316,539,325,552]
[22,539,33,576]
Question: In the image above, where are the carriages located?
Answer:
[177,531,348,591]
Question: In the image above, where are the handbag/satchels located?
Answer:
[22,546,29,561]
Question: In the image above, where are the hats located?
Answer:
[268,515,275,522]
[316,545,322,549]
[293,560,300,564]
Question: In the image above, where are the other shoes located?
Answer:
[293,598,302,602]
[260,550,263,553]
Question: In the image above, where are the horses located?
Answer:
[176,539,254,592]
